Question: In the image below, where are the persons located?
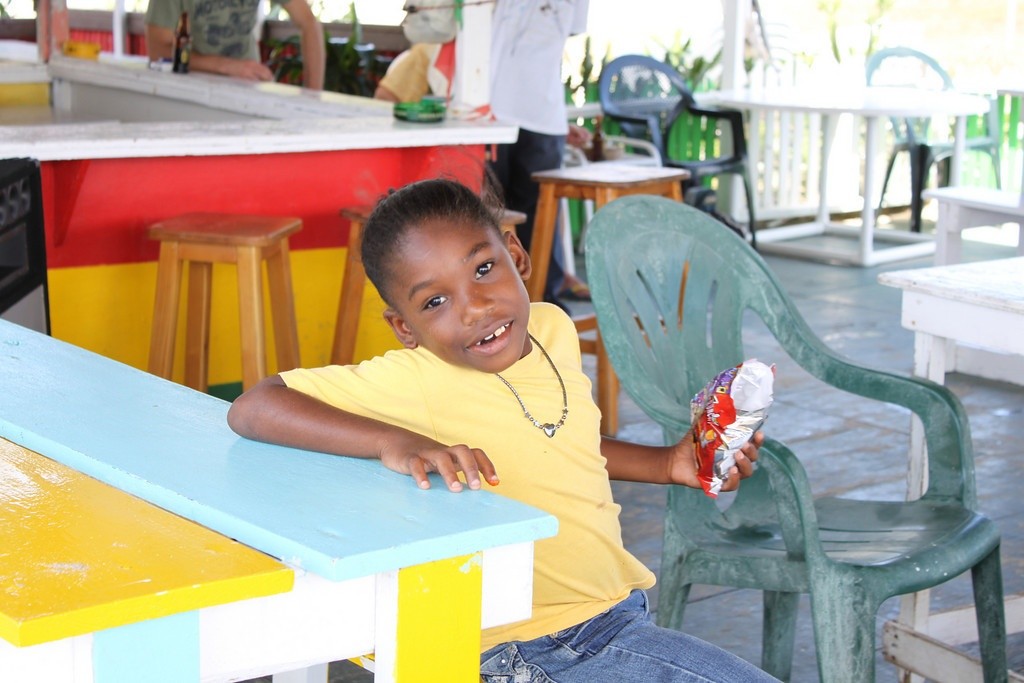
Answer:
[145,0,326,92]
[226,177,788,682]
[482,0,594,320]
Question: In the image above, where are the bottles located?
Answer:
[172,10,192,73]
[591,116,604,161]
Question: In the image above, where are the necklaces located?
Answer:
[492,331,569,438]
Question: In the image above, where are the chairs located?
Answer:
[600,53,757,245]
[583,189,1012,683]
[862,45,1004,238]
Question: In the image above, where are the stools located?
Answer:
[147,212,302,399]
[523,162,695,424]
[329,203,528,356]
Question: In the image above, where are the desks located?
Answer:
[1,315,563,683]
[730,92,992,254]
[875,256,1024,683]
[918,178,1024,266]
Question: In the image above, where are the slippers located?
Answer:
[559,284,591,302]
